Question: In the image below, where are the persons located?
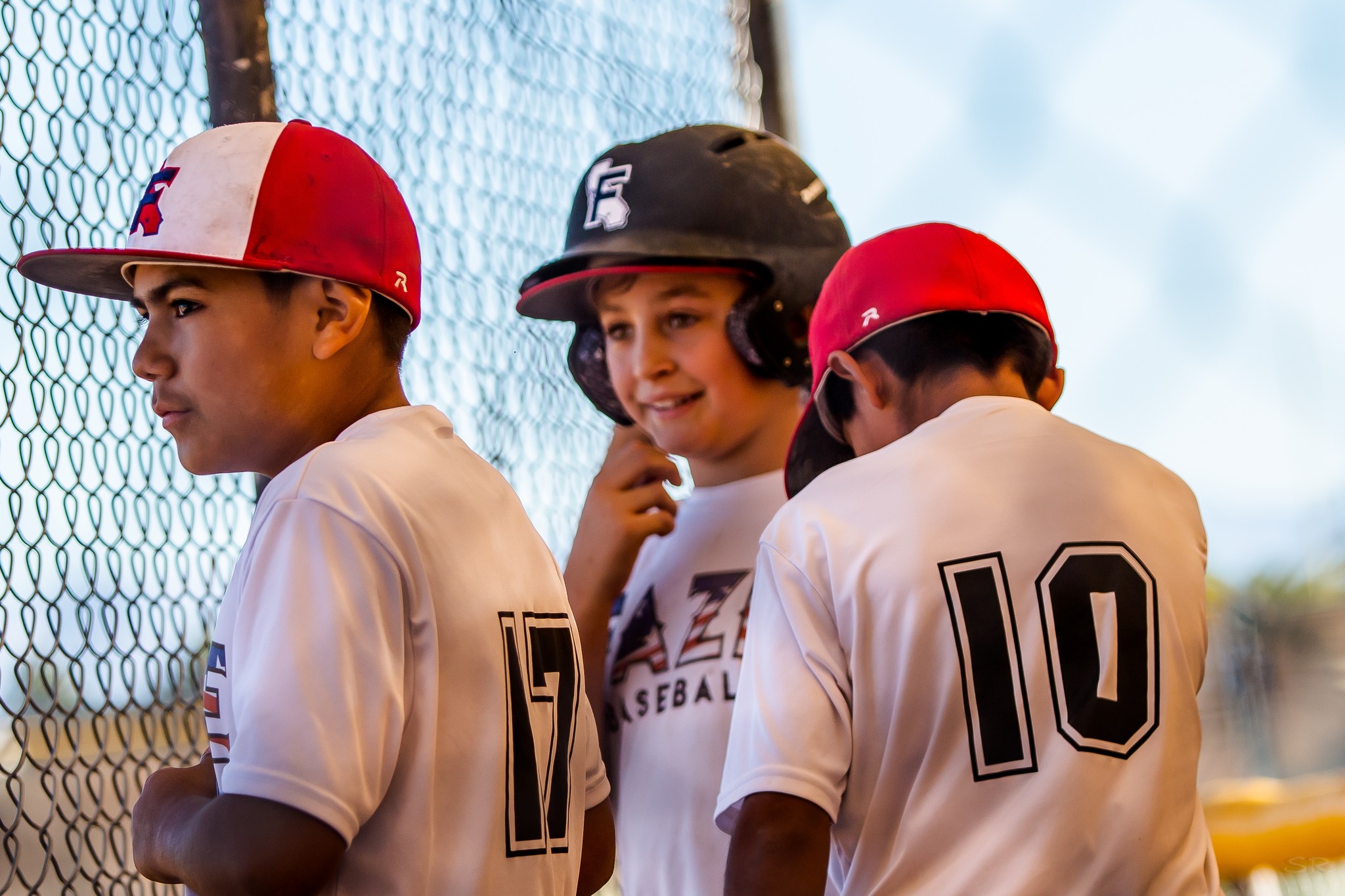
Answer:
[14,119,616,896]
[714,223,1227,896]
[513,122,850,896]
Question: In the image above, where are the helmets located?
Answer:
[516,123,853,426]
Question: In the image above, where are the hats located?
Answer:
[15,119,422,342]
[783,222,1057,500]
